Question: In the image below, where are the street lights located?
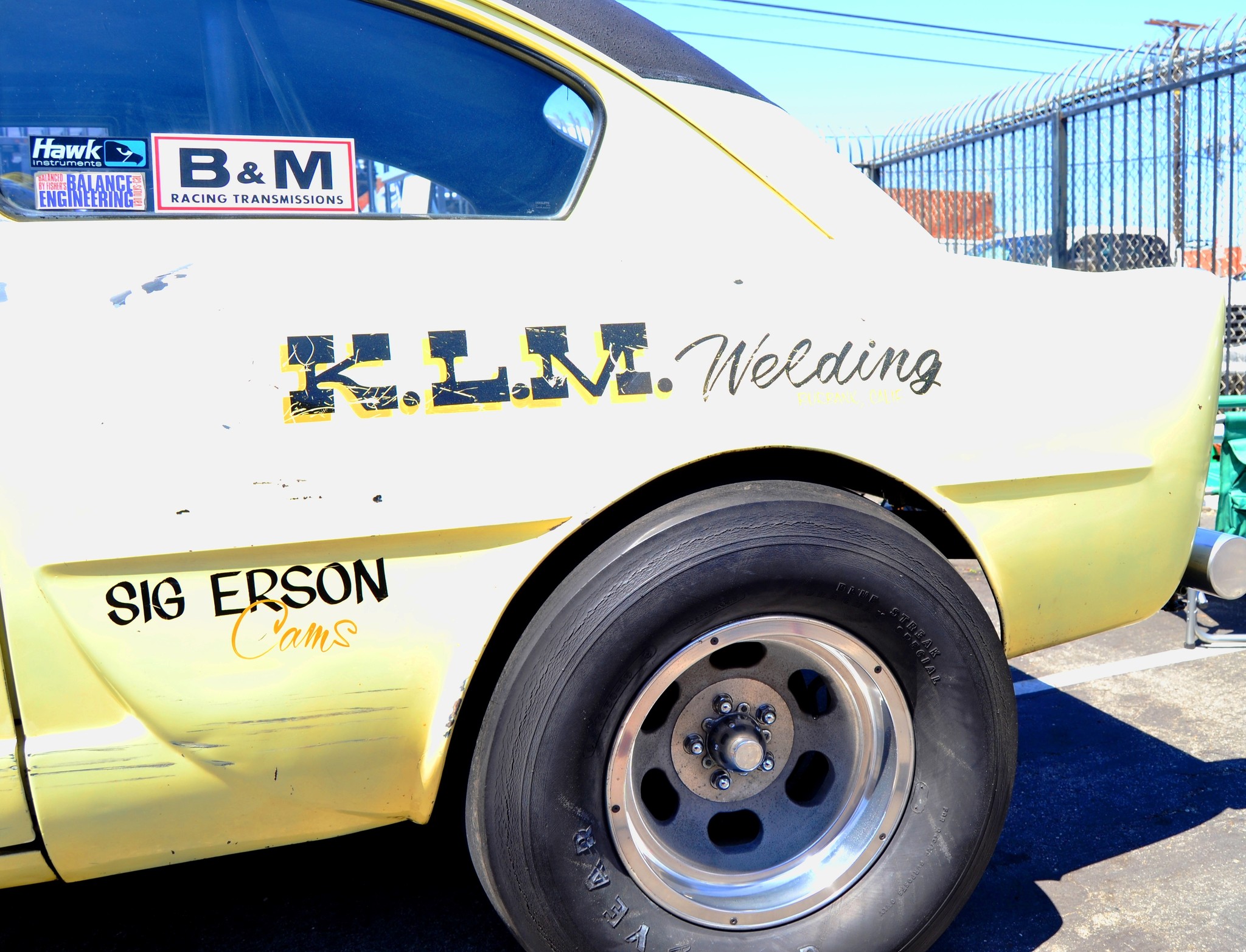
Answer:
[1146,18,1212,252]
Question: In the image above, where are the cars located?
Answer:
[0,0,1225,952]
[963,225,1246,343]
